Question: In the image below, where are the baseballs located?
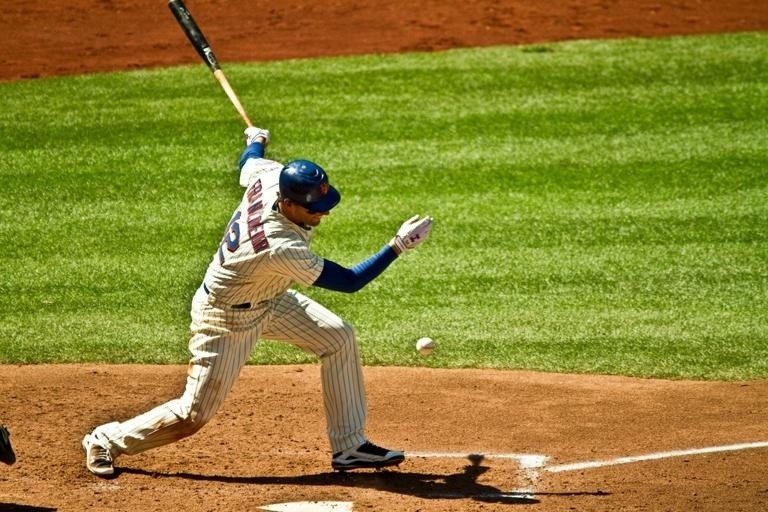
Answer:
[415,338,434,356]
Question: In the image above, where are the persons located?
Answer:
[81,126,434,476]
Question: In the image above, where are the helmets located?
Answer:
[279,159,340,212]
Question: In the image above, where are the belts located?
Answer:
[203,283,250,308]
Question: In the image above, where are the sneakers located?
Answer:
[81,433,115,475]
[332,441,405,470]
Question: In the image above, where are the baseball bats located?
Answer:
[167,1,253,127]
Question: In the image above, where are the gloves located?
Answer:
[389,214,434,254]
[244,127,271,148]
